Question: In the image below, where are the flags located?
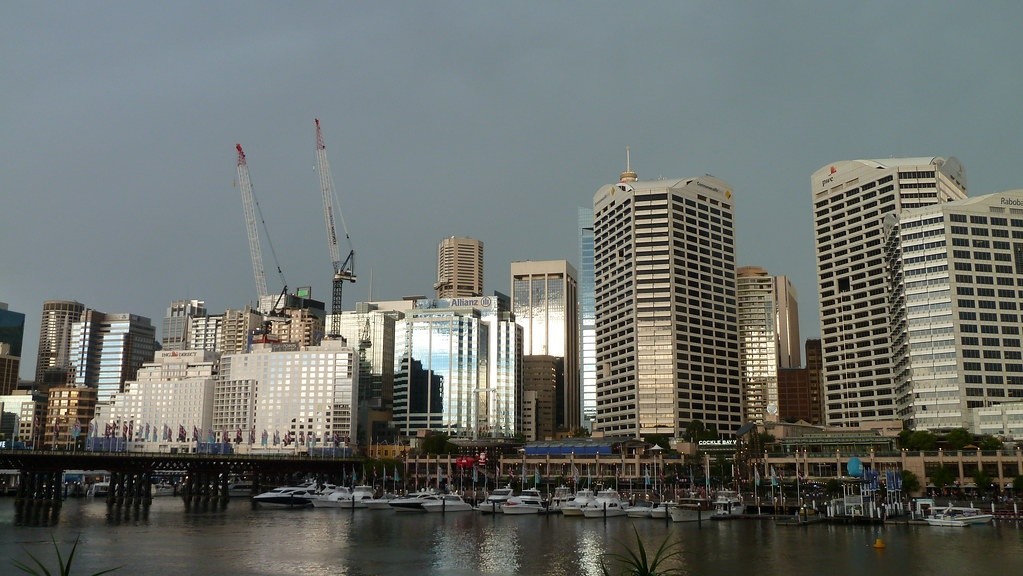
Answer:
[333,448,809,493]
[857,466,904,495]
[31,415,351,450]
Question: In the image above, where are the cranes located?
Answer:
[313,116,360,341]
[235,142,292,343]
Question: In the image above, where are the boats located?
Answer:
[419,493,473,512]
[386,491,438,512]
[925,501,995,532]
[252,480,393,511]
[476,483,519,513]
[626,485,746,523]
[500,487,549,515]
[548,484,576,513]
[580,489,627,519]
[560,490,597,516]
